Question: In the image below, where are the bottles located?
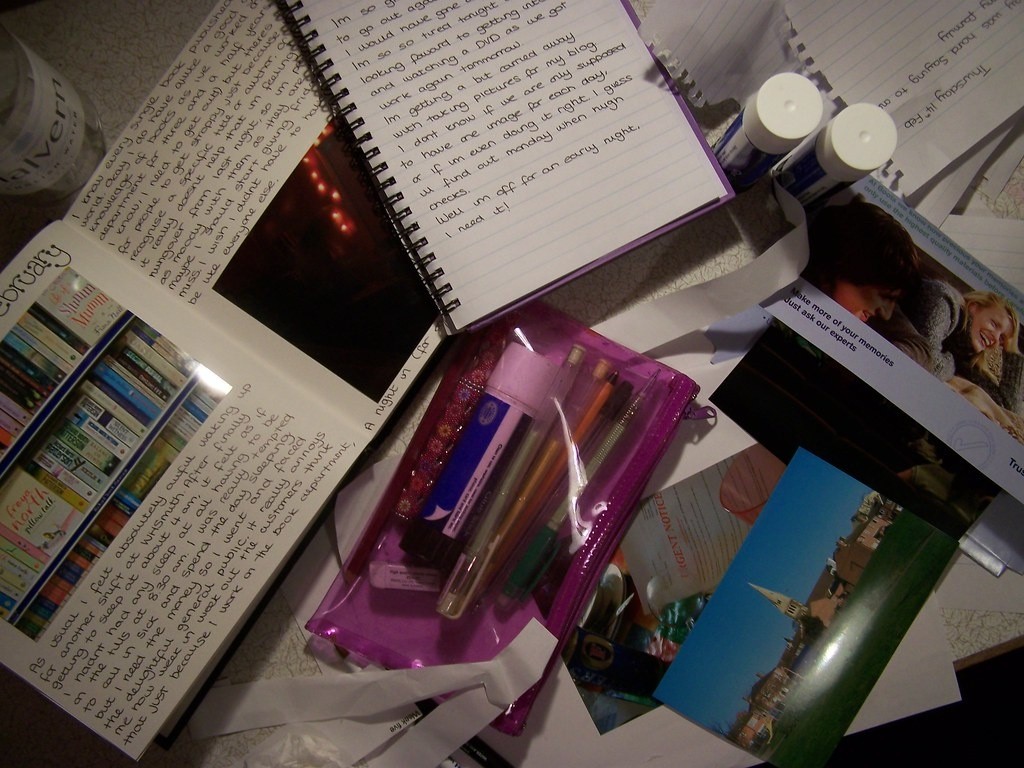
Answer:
[0,25,109,205]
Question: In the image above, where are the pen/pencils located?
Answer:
[435,345,662,622]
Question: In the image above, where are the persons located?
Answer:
[801,204,1024,521]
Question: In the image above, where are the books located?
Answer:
[0,1,451,767]
[0,267,217,641]
[273,0,735,336]
